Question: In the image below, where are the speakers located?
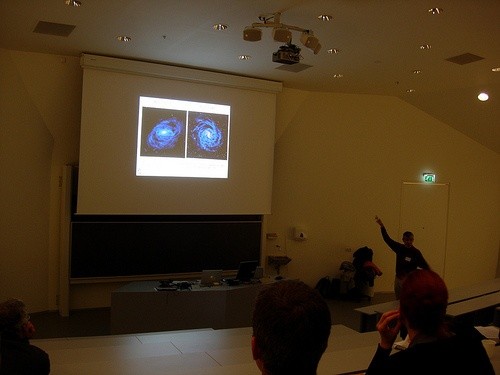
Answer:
[300,34,322,55]
[272,29,292,42]
[244,29,261,42]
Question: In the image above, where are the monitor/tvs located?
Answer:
[237,260,259,283]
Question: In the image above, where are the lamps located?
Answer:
[244,13,320,50]
[423,173,436,183]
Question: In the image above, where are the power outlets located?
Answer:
[271,240,281,251]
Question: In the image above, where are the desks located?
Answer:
[30,277,500,375]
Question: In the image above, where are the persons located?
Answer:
[251,278,331,375]
[375,216,431,300]
[0,298,51,375]
[366,269,495,375]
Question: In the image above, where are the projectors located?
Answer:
[272,50,299,64]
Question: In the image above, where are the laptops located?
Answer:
[200,270,222,287]
